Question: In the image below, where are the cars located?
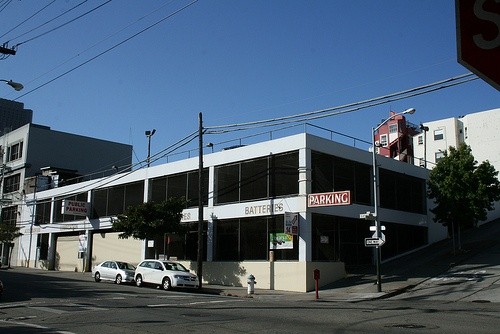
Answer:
[134,260,199,291]
[92,261,136,285]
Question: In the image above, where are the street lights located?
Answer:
[145,129,156,168]
[371,108,416,293]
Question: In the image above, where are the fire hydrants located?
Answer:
[246,274,257,294]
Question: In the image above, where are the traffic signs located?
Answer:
[365,238,384,246]
[284,212,298,235]
